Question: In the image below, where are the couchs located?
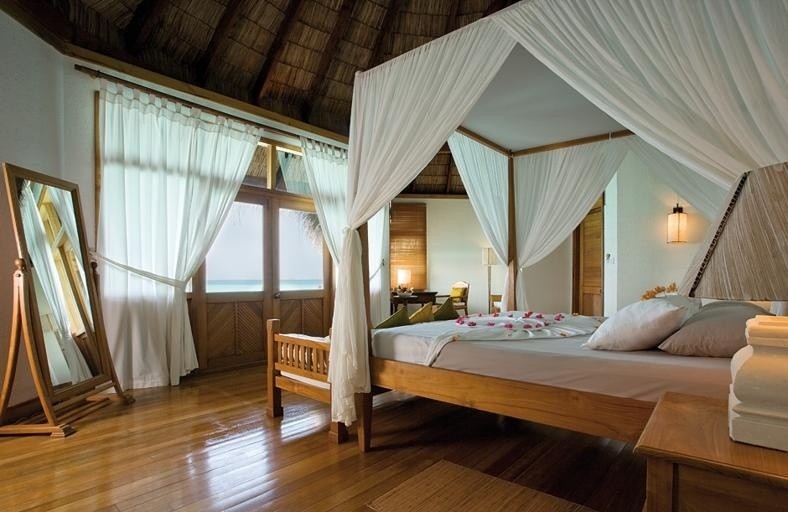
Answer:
[264,295,503,445]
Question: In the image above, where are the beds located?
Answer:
[332,0,788,512]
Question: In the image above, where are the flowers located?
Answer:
[455,305,578,328]
[638,281,681,298]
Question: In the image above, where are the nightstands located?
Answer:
[631,390,788,512]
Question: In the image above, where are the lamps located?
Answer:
[482,247,499,313]
[667,206,688,242]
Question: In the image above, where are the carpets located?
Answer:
[363,459,598,512]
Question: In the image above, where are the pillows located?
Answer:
[374,296,459,329]
[658,299,777,357]
[580,296,703,351]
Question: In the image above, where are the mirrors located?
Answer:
[1,162,113,405]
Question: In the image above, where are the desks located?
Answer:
[389,292,438,317]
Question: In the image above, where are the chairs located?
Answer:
[431,280,470,315]
